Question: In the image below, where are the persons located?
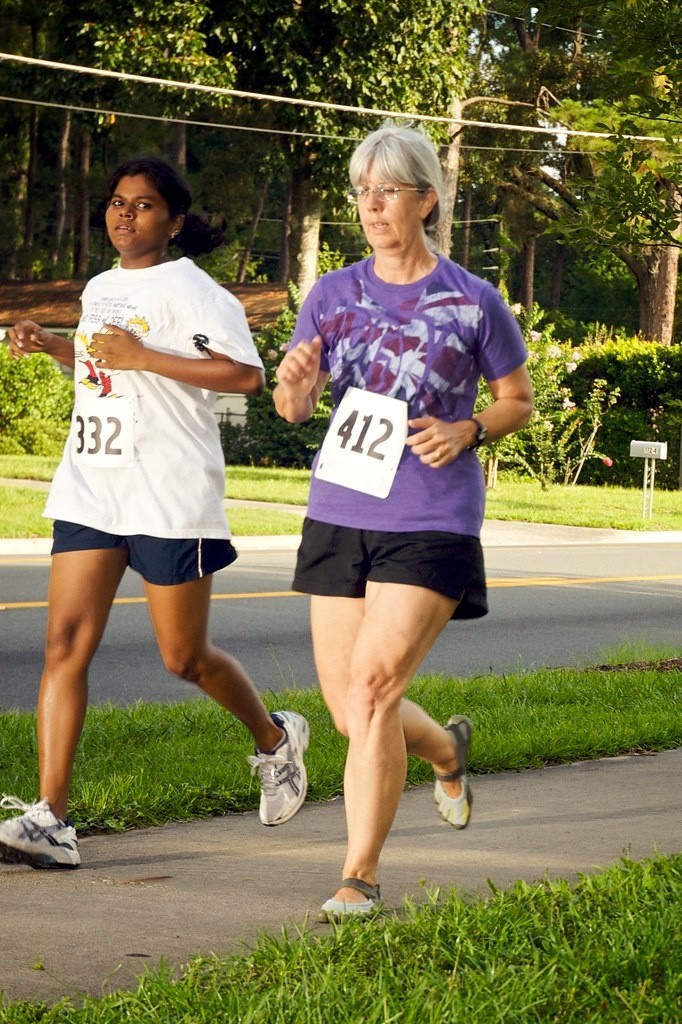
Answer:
[272,119,535,925]
[0,155,311,871]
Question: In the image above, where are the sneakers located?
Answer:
[246,710,310,827]
[0,794,82,871]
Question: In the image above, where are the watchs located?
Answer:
[467,417,488,451]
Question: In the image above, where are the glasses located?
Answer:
[345,182,426,204]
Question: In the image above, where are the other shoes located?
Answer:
[432,715,475,831]
[316,877,384,924]
